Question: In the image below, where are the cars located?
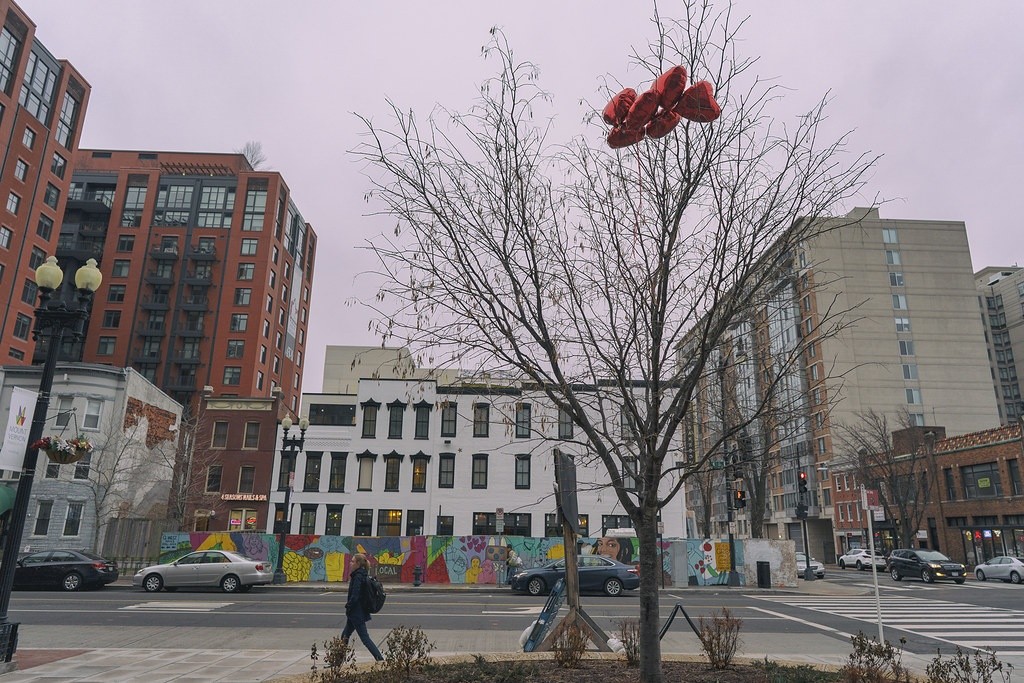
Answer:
[974,555,1024,584]
[794,551,826,579]
[134,549,274,594]
[888,548,966,584]
[10,546,120,592]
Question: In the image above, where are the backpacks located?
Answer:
[365,576,386,614]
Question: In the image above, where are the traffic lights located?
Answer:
[797,470,808,494]
[734,490,748,509]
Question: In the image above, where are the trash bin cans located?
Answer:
[756,560,771,588]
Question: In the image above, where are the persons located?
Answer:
[503,544,519,585]
[337,553,386,661]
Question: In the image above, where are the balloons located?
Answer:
[599,65,722,149]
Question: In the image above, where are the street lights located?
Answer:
[271,410,309,585]
[0,249,102,665]
[718,337,748,587]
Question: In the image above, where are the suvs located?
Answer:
[839,548,887,572]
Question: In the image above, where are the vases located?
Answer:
[47,450,84,464]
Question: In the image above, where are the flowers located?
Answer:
[31,434,93,455]
